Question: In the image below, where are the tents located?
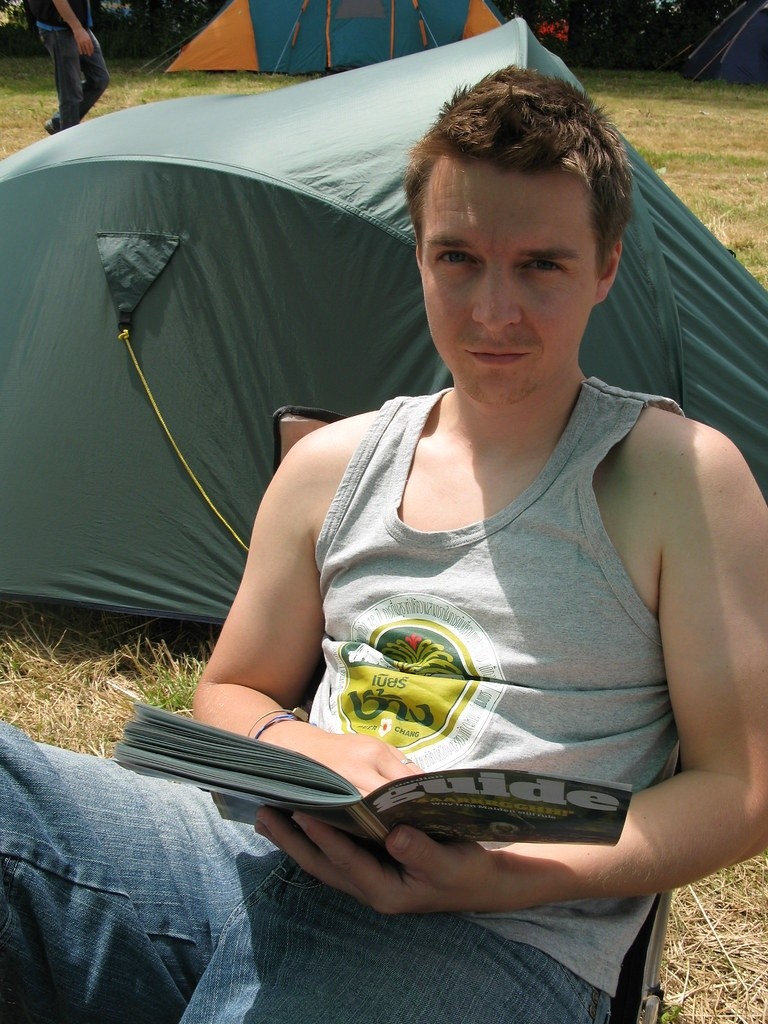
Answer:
[0,0,768,630]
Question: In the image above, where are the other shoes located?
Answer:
[44,119,57,135]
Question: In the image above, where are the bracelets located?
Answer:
[246,705,311,741]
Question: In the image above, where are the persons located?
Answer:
[36,0,110,135]
[0,66,768,1024]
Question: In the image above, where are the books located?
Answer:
[115,701,631,870]
